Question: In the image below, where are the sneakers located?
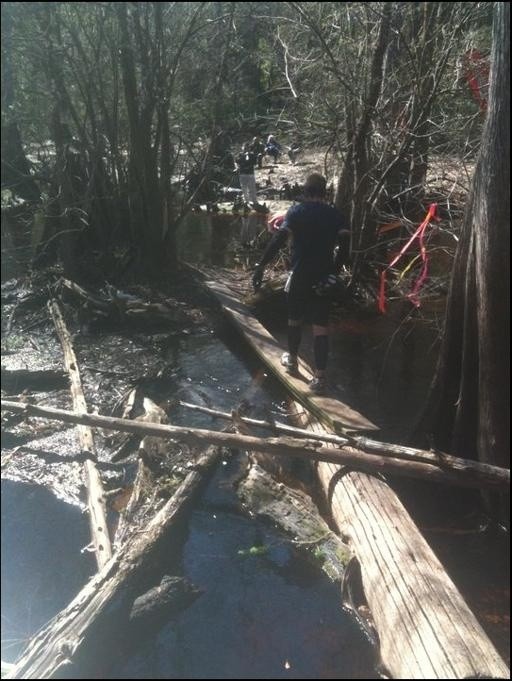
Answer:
[309,376,327,396]
[280,351,299,374]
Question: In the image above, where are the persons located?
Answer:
[252,173,350,395]
[218,134,304,206]
